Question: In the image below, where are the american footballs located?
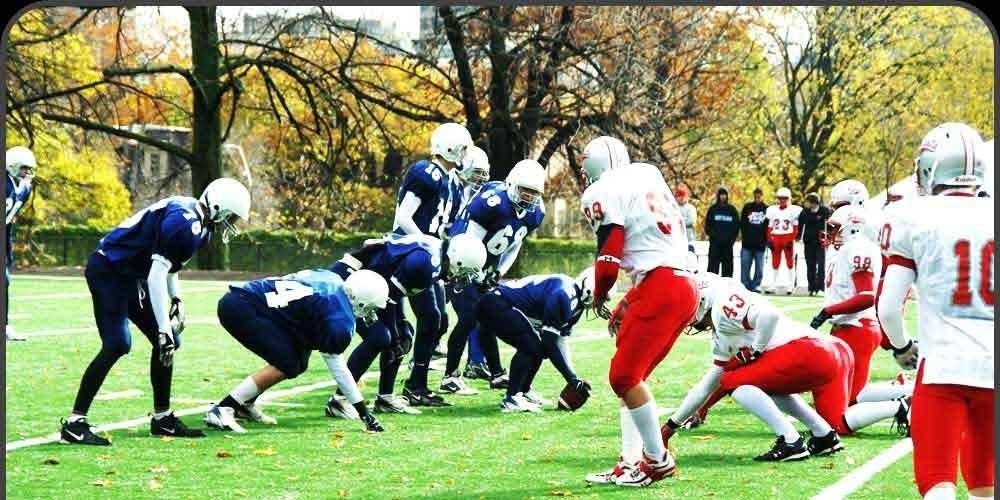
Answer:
[558,382,588,410]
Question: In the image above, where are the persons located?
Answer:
[203,120,615,432]
[6,147,37,341]
[59,177,251,446]
[581,136,702,486]
[660,121,994,500]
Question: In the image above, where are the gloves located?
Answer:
[157,331,176,369]
[397,318,414,353]
[169,297,186,338]
[785,241,792,250]
[569,378,591,397]
[361,413,384,432]
[810,308,832,329]
[660,423,675,450]
[894,339,919,370]
[679,410,702,430]
[766,241,774,251]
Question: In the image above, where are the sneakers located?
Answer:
[751,285,825,297]
[615,448,676,487]
[438,360,556,413]
[373,394,423,415]
[807,428,844,457]
[5,325,27,341]
[201,402,249,434]
[431,351,447,360]
[753,432,811,462]
[408,358,415,369]
[58,417,109,445]
[403,380,454,406]
[236,400,277,425]
[324,393,362,419]
[890,373,914,437]
[585,456,640,484]
[147,411,202,437]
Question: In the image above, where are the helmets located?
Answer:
[818,205,865,249]
[775,186,792,208]
[6,146,37,188]
[579,136,630,189]
[683,271,722,336]
[444,233,487,292]
[342,269,389,329]
[575,265,619,322]
[913,122,986,197]
[431,122,546,214]
[199,177,252,245]
[828,180,870,210]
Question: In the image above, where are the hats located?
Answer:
[674,183,688,198]
